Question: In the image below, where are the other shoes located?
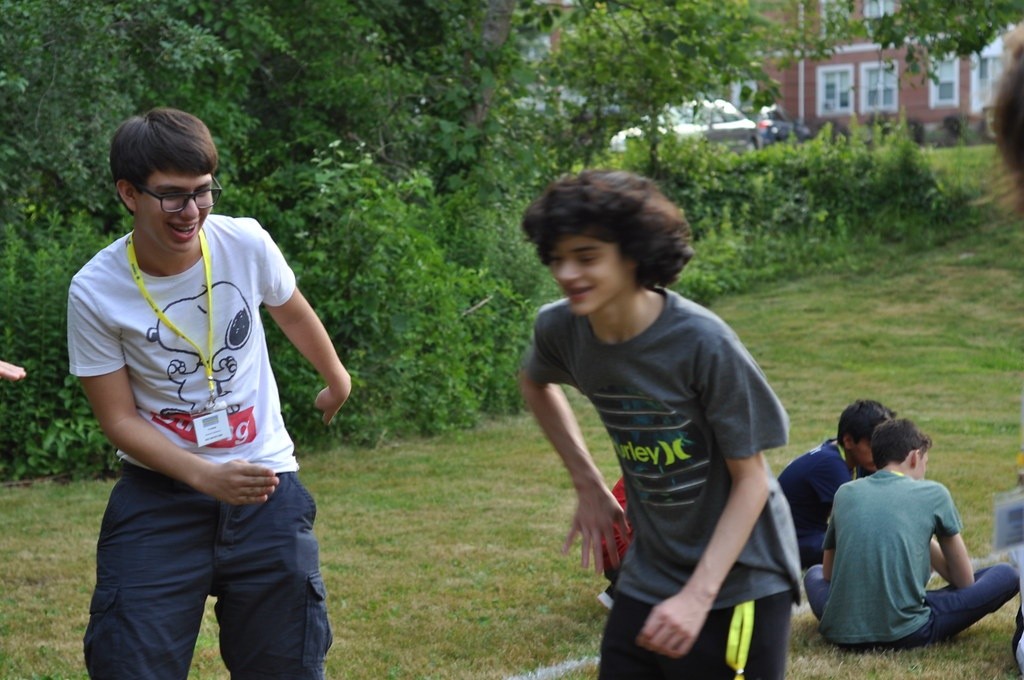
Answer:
[599,592,614,612]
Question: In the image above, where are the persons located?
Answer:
[522,168,804,680]
[0,360,27,381]
[66,109,354,679]
[778,397,1021,654]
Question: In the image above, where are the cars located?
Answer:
[610,96,758,163]
[743,102,815,152]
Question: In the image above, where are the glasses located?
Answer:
[132,175,223,214]
